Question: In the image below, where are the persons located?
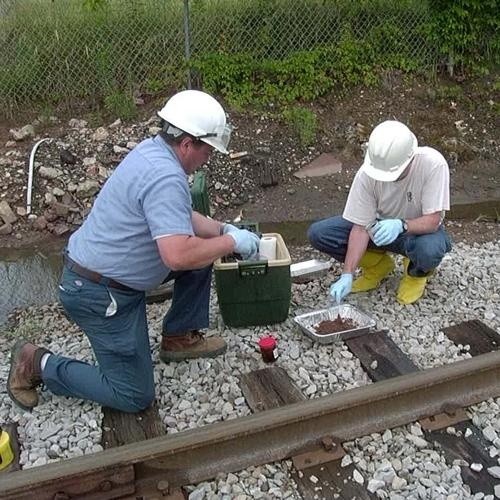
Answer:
[306,118,454,308]
[5,88,262,415]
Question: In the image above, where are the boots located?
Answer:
[353,251,395,293]
[397,256,435,305]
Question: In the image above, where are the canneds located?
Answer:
[259,337,279,363]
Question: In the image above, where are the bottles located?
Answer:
[364,220,384,247]
[259,337,277,365]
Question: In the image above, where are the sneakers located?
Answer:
[7,340,53,409]
[160,330,227,362]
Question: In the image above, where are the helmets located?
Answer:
[157,89,232,154]
[363,120,418,182]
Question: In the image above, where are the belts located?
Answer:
[62,253,134,291]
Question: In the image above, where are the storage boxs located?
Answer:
[209,232,294,328]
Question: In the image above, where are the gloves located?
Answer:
[369,219,403,246]
[223,224,260,260]
[331,274,353,303]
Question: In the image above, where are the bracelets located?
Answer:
[219,222,227,237]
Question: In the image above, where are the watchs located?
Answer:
[398,218,409,236]
